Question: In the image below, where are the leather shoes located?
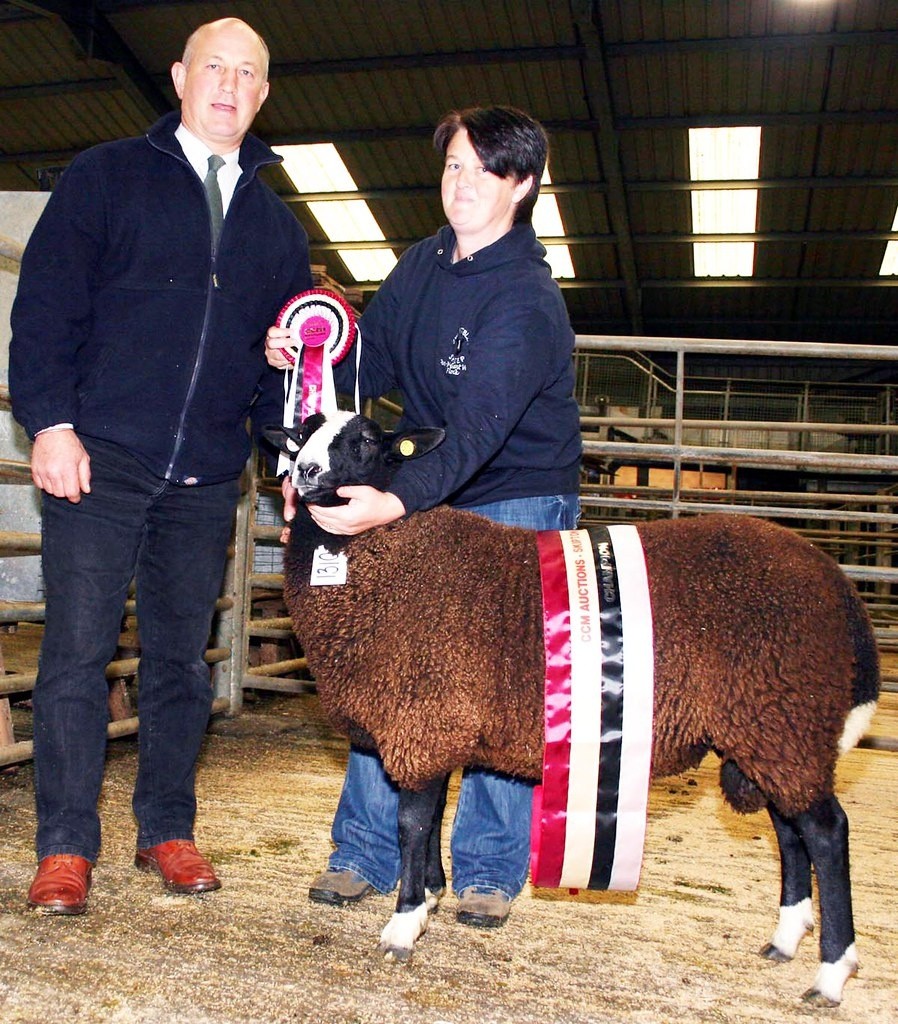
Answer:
[134,839,221,893]
[26,853,92,915]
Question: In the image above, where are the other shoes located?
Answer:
[456,886,510,928]
[308,865,374,905]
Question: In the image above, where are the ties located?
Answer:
[204,155,226,260]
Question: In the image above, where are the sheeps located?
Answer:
[255,406,882,1010]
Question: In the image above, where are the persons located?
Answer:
[8,16,315,915]
[265,103,579,928]
